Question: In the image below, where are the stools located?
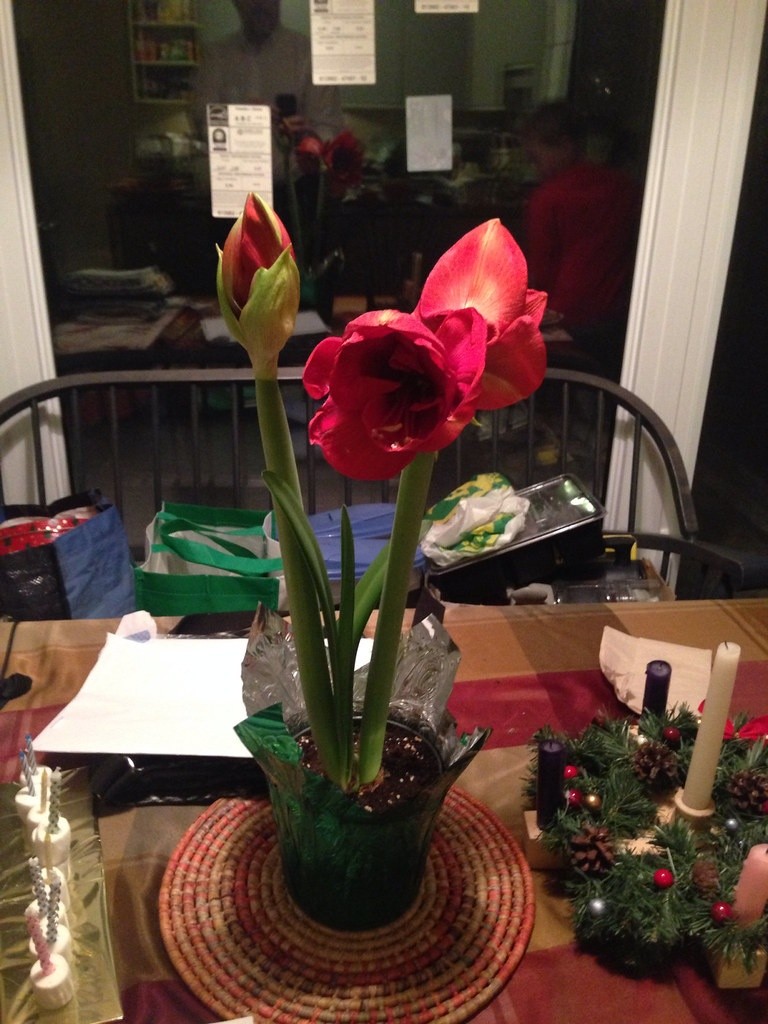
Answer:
[520,332,612,502]
[203,311,331,516]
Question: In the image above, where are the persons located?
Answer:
[189,0,343,422]
[519,101,638,330]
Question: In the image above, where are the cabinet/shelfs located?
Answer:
[122,0,207,104]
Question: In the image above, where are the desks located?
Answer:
[0,599,768,1024]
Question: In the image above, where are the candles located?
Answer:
[536,740,565,829]
[642,660,672,719]
[732,844,768,926]
[683,640,740,809]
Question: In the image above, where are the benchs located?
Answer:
[0,363,768,622]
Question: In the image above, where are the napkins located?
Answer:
[599,624,712,718]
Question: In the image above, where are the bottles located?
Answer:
[133,0,201,99]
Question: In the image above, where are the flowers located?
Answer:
[293,132,366,262]
[214,194,549,784]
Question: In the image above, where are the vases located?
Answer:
[234,595,492,933]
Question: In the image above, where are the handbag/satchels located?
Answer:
[0,491,133,620]
[132,500,289,612]
